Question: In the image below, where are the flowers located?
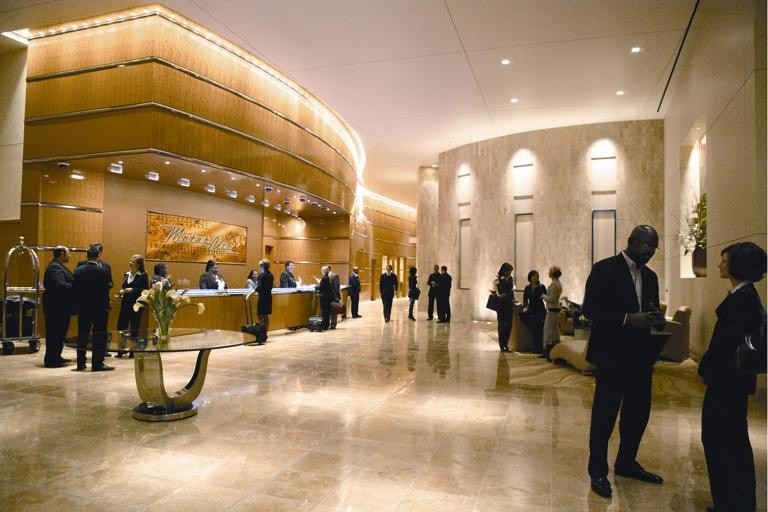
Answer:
[672,192,707,256]
[133,274,206,327]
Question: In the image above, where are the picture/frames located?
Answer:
[145,209,248,266]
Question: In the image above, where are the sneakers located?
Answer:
[75,362,87,371]
[90,361,118,372]
[42,355,73,370]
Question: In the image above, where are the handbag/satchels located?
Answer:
[328,300,346,315]
[486,293,509,313]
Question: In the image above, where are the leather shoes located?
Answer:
[590,472,614,501]
[614,461,665,486]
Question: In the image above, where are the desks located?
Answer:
[61,328,257,422]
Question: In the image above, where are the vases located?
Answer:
[691,245,707,278]
[155,327,173,345]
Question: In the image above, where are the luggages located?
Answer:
[308,287,325,332]
[241,298,268,346]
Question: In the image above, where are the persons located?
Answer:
[540,266,563,360]
[580,224,663,497]
[379,264,397,322]
[349,266,362,318]
[408,266,418,321]
[436,265,452,323]
[520,270,548,355]
[697,240,767,512]
[42,243,339,373]
[427,264,441,320]
[492,262,515,353]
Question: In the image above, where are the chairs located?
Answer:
[506,300,692,377]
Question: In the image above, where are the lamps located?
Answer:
[105,160,336,229]
